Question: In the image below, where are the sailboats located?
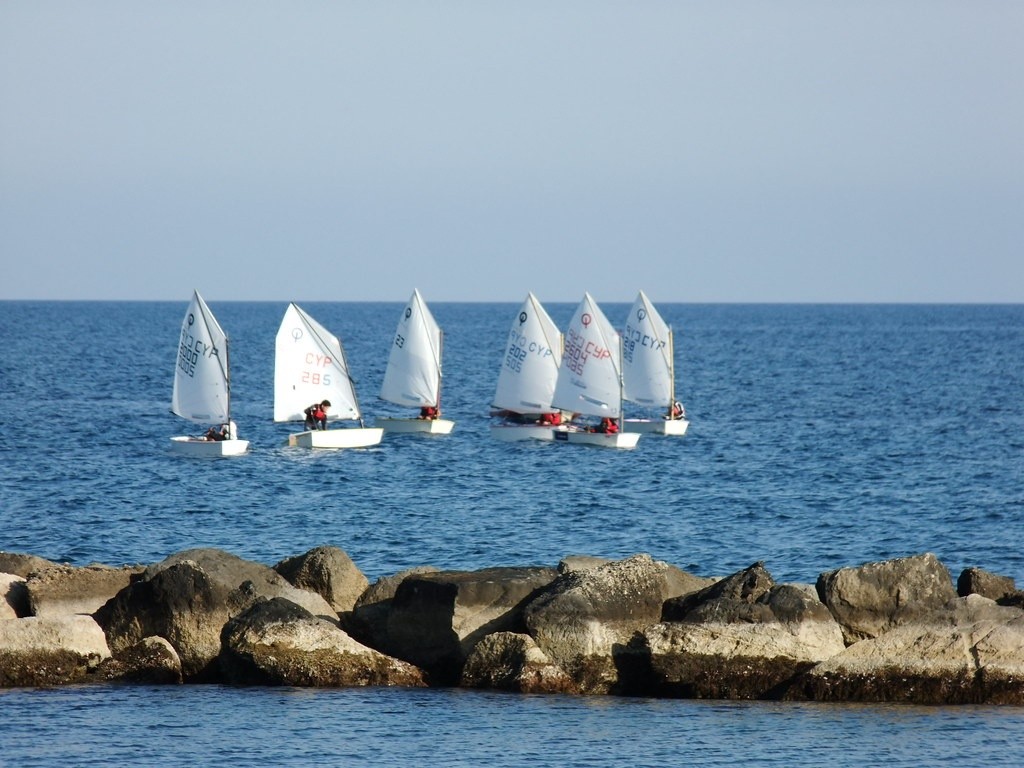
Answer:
[380,287,455,436]
[620,290,692,438]
[166,286,251,457]
[549,292,642,452]
[272,300,385,450]
[491,289,577,442]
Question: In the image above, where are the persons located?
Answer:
[663,401,684,420]
[536,413,561,425]
[583,417,619,434]
[304,400,331,432]
[418,406,441,419]
[206,420,238,441]
